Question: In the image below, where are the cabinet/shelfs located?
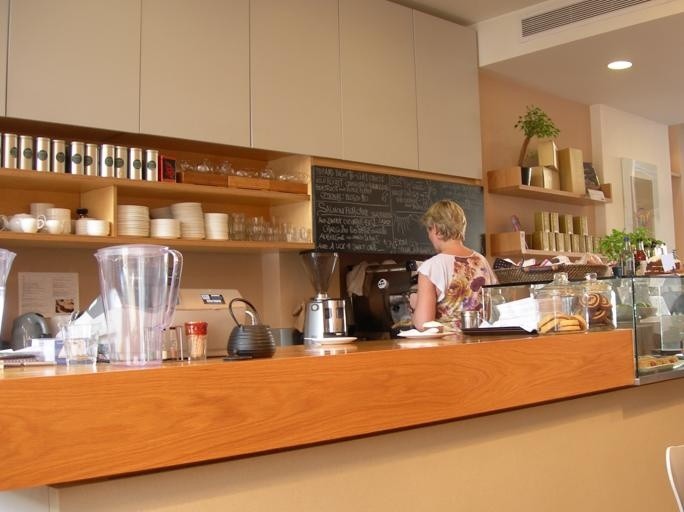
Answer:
[486,166,612,260]
[1,113,312,350]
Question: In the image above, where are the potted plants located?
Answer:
[514,104,560,187]
[593,226,662,278]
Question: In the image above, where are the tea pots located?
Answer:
[92,244,183,366]
[227,297,277,358]
[0,212,46,233]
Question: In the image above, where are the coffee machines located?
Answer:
[359,263,418,339]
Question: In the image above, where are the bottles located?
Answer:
[116,145,127,178]
[86,144,99,176]
[635,238,648,277]
[577,272,618,330]
[535,270,590,336]
[52,140,65,173]
[70,141,84,176]
[461,310,478,330]
[37,137,50,171]
[645,241,667,257]
[3,133,16,169]
[130,148,142,181]
[100,145,115,177]
[19,135,33,170]
[147,150,158,181]
[621,237,635,277]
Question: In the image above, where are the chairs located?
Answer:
[663,443,683,512]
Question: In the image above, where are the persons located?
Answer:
[164,159,175,180]
[409,200,506,332]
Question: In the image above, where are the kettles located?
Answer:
[11,312,51,351]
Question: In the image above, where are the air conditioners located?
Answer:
[519,0,656,40]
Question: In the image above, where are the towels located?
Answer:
[345,259,369,298]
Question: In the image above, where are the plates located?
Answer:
[29,203,55,219]
[205,212,230,240]
[304,336,358,346]
[118,204,149,237]
[150,202,205,240]
[46,208,71,234]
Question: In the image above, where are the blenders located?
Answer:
[291,251,348,345]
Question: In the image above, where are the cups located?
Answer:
[232,211,310,241]
[46,220,61,234]
[87,221,110,236]
[162,325,183,364]
[187,154,308,183]
[19,218,44,233]
[61,322,99,366]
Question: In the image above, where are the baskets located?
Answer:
[491,264,609,284]
